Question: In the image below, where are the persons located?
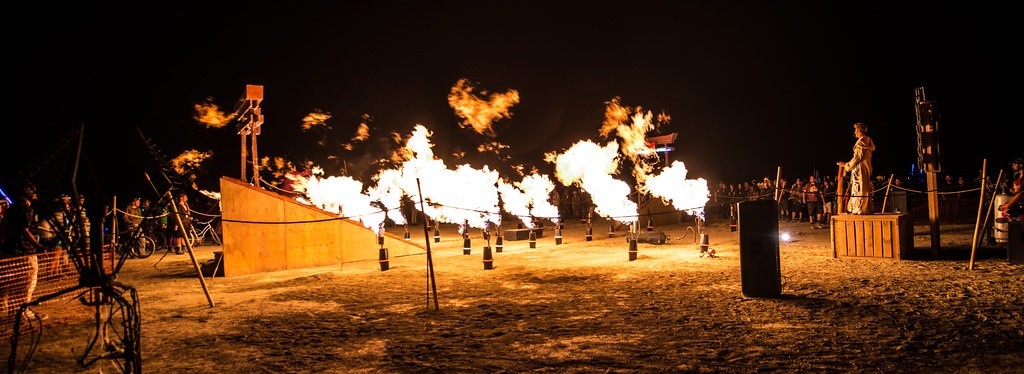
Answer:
[838,122,879,215]
[549,186,586,222]
[1,180,197,321]
[714,160,1024,268]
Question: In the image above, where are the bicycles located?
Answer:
[103,219,167,259]
[173,215,222,250]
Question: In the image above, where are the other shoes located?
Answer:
[20,311,34,321]
[169,248,189,254]
